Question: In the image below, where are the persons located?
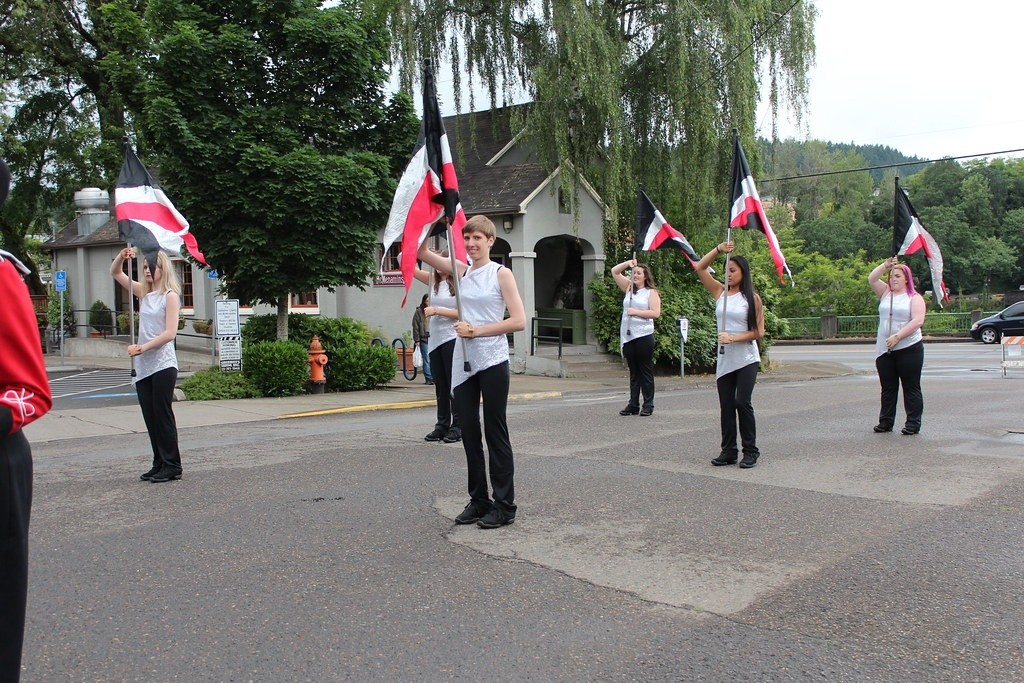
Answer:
[396,248,461,442]
[868,255,927,434]
[610,259,661,416]
[417,211,526,528]
[0,158,54,683]
[111,247,183,482]
[696,241,764,467]
[412,293,434,385]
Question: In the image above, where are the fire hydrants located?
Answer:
[306,334,328,394]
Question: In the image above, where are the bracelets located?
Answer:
[717,247,720,253]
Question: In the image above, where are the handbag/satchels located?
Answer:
[412,346,423,367]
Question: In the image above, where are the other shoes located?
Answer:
[901,428,919,435]
[426,381,434,385]
[873,426,893,433]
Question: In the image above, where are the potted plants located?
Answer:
[89,300,112,337]
[193,320,213,335]
[397,330,415,371]
[117,310,139,334]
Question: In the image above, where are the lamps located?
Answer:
[504,215,514,229]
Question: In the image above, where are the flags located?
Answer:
[380,66,467,308]
[892,177,950,307]
[633,191,716,274]
[727,135,794,287]
[114,143,211,282]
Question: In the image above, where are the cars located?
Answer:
[969,300,1024,345]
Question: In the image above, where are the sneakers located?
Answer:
[476,499,517,529]
[140,462,182,482]
[711,454,738,466]
[739,452,760,468]
[455,499,493,525]
[619,406,640,416]
[424,429,448,441]
[641,407,653,416]
[443,426,461,443]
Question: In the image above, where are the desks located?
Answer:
[537,307,586,345]
